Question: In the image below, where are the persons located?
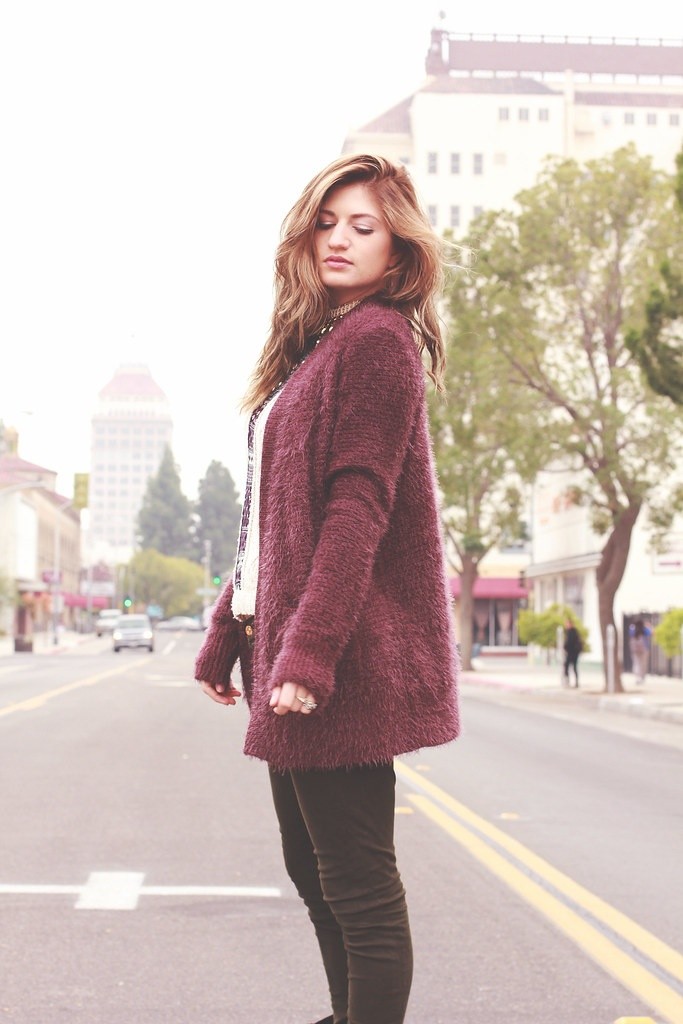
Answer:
[561,621,581,682]
[192,151,461,1024]
[627,618,653,683]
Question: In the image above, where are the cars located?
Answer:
[156,615,200,632]
[113,614,153,653]
[96,609,121,636]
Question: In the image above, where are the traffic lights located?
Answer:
[213,575,221,586]
[124,597,132,608]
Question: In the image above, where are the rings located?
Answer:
[295,696,306,704]
[305,702,317,710]
